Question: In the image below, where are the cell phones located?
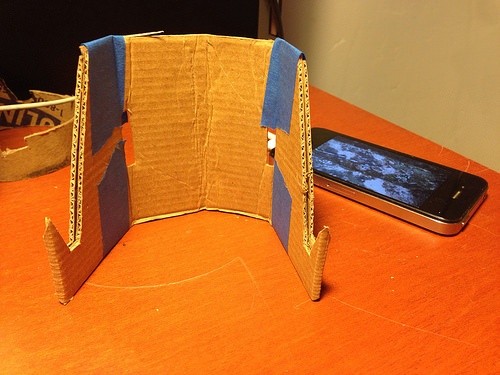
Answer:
[310,127,488,237]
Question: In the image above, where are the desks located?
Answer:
[0,85,500,374]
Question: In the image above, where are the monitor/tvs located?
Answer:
[0,0,270,101]
